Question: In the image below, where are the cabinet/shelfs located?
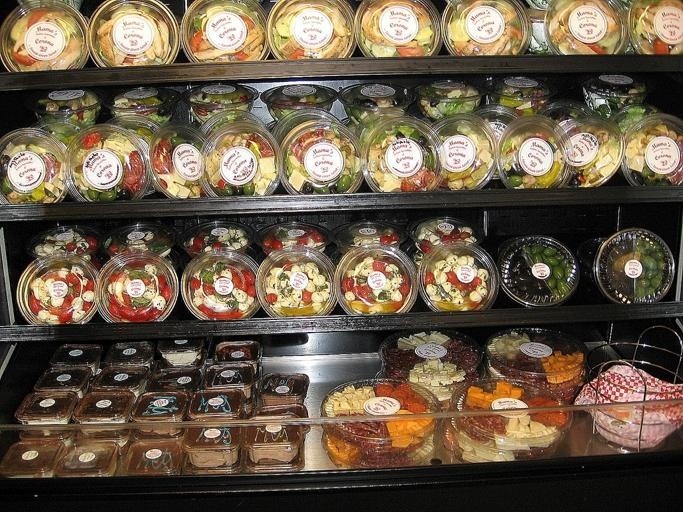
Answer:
[0,54,683,512]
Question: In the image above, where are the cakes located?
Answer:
[322,331,585,470]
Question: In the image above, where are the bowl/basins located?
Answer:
[1,76,682,204]
[15,217,676,323]
[1,1,682,73]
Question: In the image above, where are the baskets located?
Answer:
[585,327,683,455]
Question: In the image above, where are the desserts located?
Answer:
[0,336,310,479]
